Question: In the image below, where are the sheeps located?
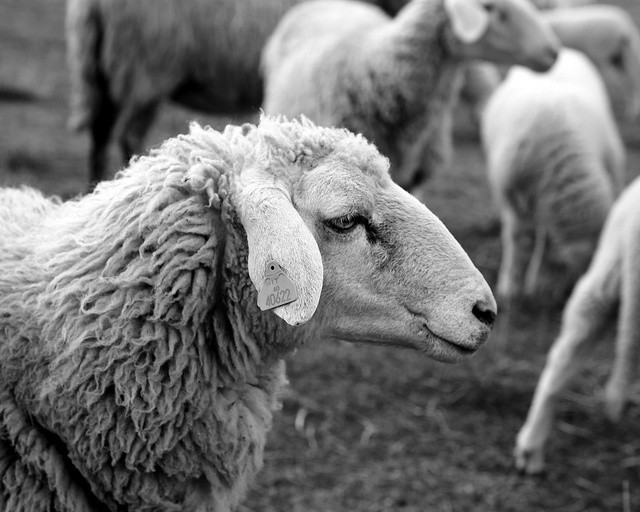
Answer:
[476,46,630,317]
[62,0,413,196]
[258,0,564,201]
[0,107,498,512]
[457,4,639,145]
[512,174,639,477]
